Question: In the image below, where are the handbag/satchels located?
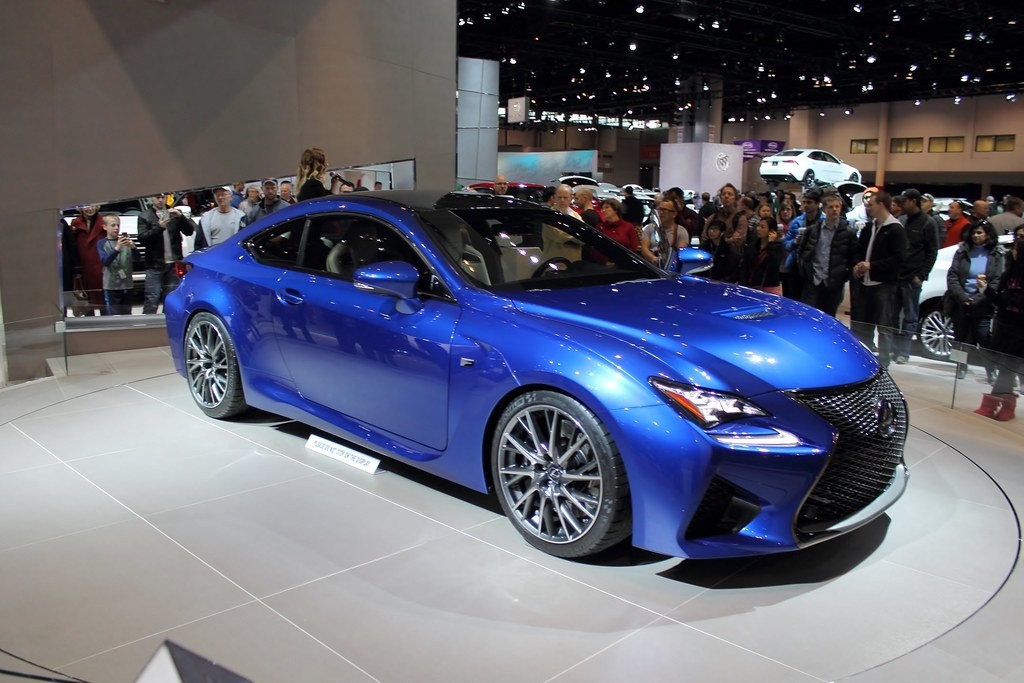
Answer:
[72,278,91,317]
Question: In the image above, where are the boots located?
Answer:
[993,392,1019,420]
[976,393,1003,417]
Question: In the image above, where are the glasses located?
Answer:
[657,207,675,213]
[863,194,871,199]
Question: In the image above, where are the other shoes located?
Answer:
[987,370,997,382]
[957,368,967,379]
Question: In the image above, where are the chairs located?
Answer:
[325,221,381,278]
[434,214,493,286]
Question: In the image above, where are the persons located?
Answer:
[61,147,383,319]
[493,175,508,196]
[523,182,1024,421]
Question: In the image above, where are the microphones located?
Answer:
[330,172,351,187]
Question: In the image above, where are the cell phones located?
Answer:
[122,232,128,243]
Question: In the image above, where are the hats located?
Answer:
[920,192,934,204]
[802,190,820,204]
[895,188,920,201]
[862,187,879,201]
[261,177,278,187]
[213,186,232,194]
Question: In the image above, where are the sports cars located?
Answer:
[161,187,912,562]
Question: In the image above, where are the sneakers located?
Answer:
[890,353,908,364]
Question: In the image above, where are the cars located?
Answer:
[757,147,862,190]
[902,233,1016,360]
[467,175,703,232]
